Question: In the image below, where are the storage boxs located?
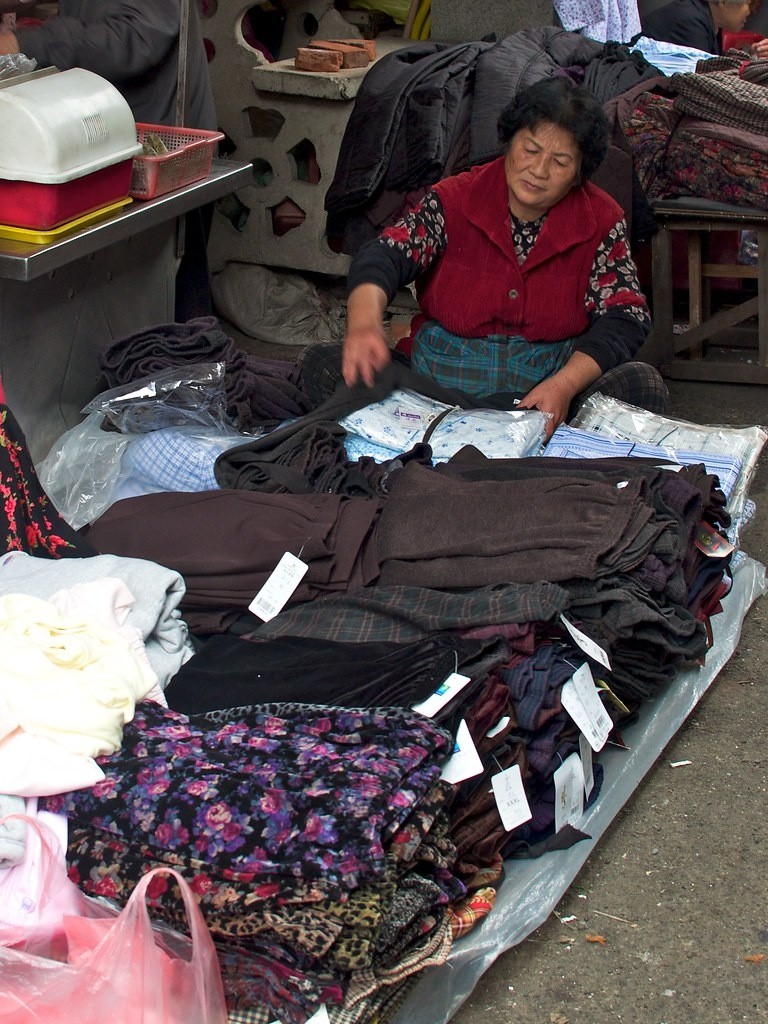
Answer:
[131,122,226,199]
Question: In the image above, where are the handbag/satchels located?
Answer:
[0,814,230,1024]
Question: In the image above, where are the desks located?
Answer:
[654,196,768,388]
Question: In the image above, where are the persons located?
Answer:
[296,76,670,443]
[644,0,768,59]
[0,0,217,323]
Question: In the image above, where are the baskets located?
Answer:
[131,122,225,200]
